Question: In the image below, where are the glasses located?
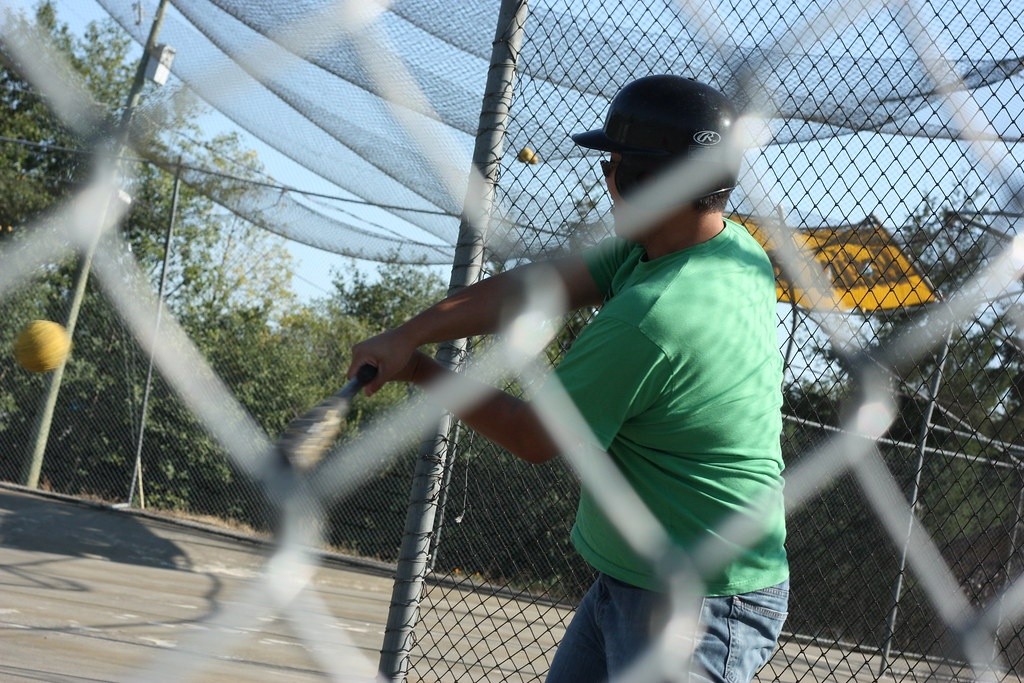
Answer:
[600,159,618,177]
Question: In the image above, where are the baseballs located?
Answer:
[13,319,75,374]
[518,147,538,165]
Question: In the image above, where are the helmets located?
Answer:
[570,75,740,201]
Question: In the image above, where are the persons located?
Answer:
[349,75,791,683]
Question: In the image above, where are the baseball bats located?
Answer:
[271,363,379,478]
